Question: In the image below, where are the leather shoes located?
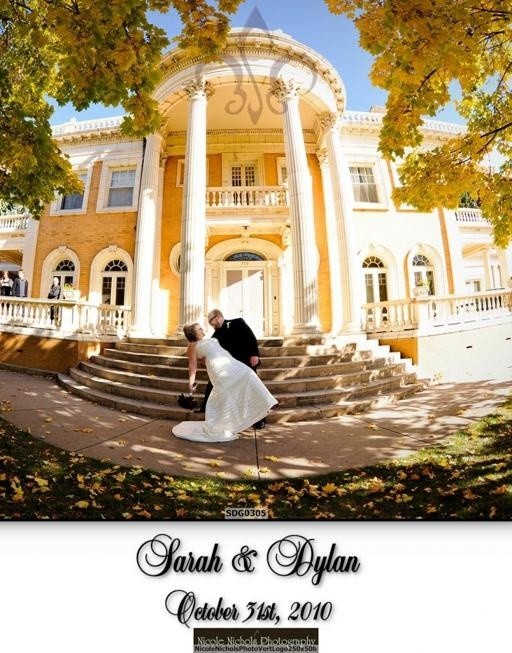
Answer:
[256,421,265,429]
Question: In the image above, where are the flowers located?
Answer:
[63,284,80,290]
[416,280,429,288]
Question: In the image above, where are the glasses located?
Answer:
[208,315,217,323]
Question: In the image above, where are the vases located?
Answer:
[63,290,80,301]
[414,287,428,297]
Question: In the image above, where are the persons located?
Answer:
[47,277,61,324]
[171,322,279,443]
[0,269,14,309]
[193,310,266,429]
[11,269,29,297]
